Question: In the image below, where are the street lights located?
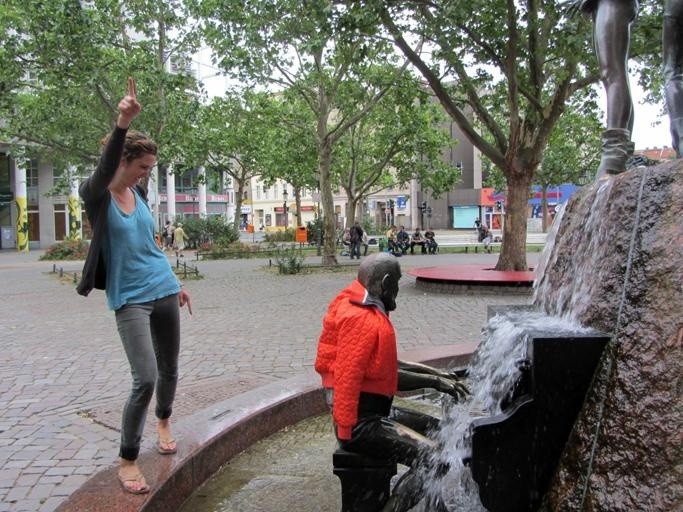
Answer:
[188,184,198,218]
[282,189,288,232]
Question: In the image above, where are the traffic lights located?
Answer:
[496,200,501,209]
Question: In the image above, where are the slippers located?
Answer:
[153,437,178,454]
[116,470,150,494]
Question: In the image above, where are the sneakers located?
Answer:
[180,254,184,257]
[175,250,178,258]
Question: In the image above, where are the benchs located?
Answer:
[345,233,549,253]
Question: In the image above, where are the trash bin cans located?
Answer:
[295,226,307,242]
[248,225,254,233]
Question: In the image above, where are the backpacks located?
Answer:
[162,226,169,237]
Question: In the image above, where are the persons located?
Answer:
[425,227,438,255]
[410,228,428,256]
[164,220,176,251]
[474,219,494,255]
[314,252,475,512]
[349,221,365,260]
[342,228,357,255]
[555,0,683,179]
[397,226,412,255]
[387,226,399,256]
[172,222,191,258]
[72,76,194,495]
[360,227,369,255]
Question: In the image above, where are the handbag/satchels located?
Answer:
[369,239,376,244]
[172,239,178,249]
